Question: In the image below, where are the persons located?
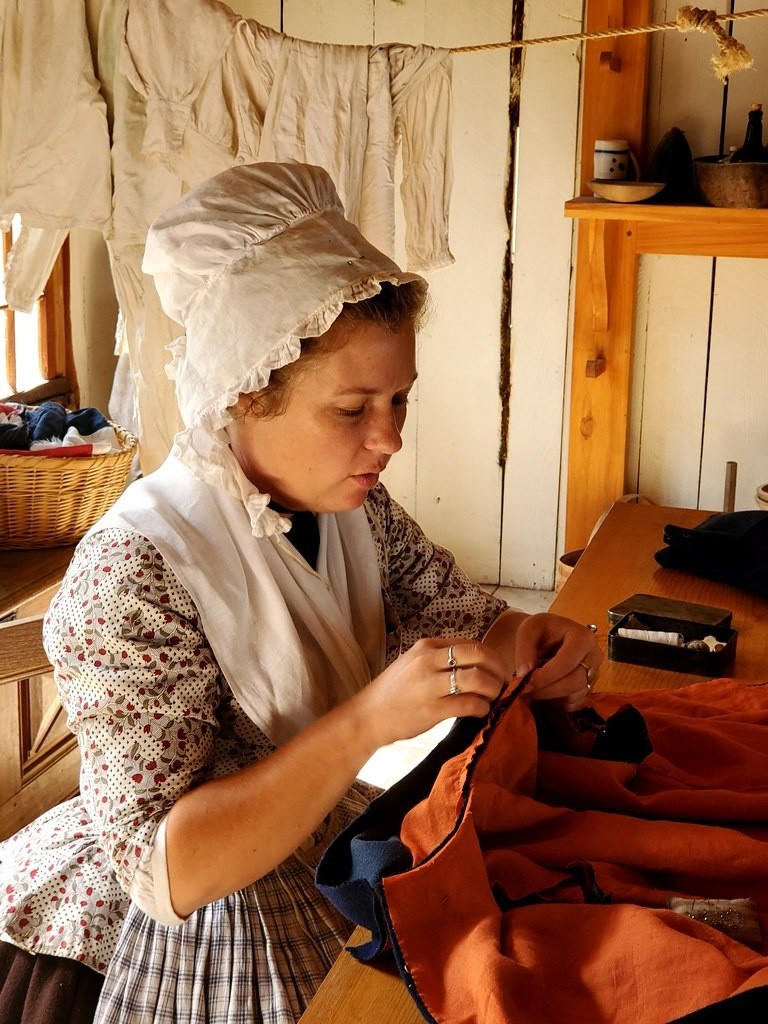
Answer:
[43,162,606,1024]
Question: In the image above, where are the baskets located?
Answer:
[0,402,138,550]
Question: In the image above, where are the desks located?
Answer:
[293,496,768,1024]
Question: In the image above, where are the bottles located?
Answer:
[723,102,768,162]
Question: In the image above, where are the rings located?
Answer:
[449,669,461,694]
[580,659,595,684]
[587,685,591,695]
[447,645,457,667]
[586,624,597,633]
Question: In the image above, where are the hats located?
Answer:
[140,160,429,536]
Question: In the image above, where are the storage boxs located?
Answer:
[609,593,739,678]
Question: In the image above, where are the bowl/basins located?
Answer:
[584,179,666,203]
[693,155,768,209]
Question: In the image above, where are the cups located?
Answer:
[593,138,628,198]
